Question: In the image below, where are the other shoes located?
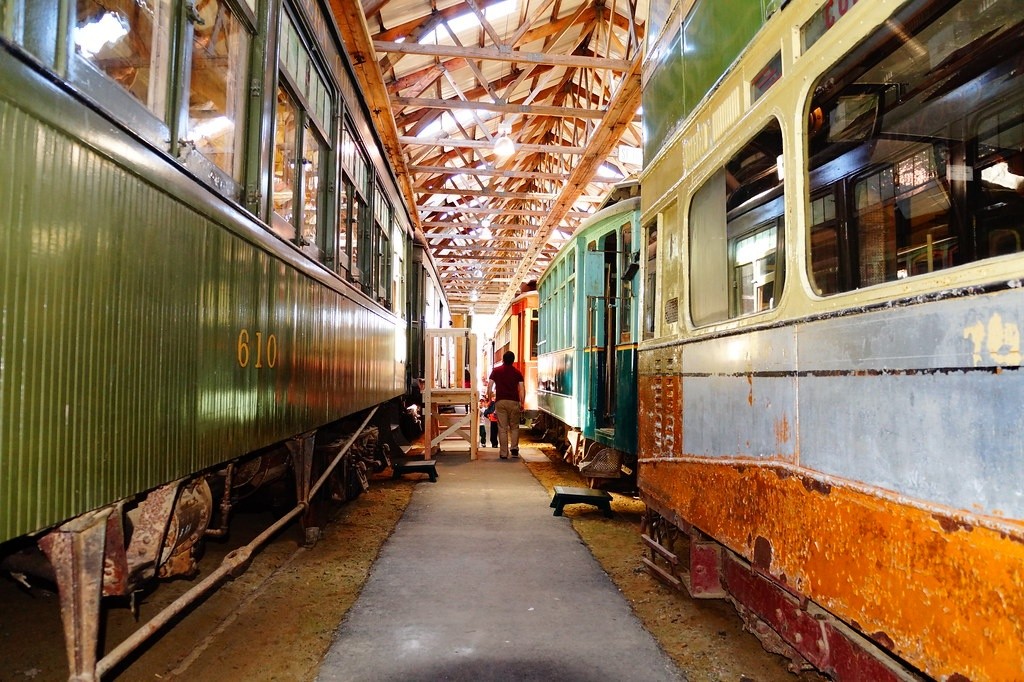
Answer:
[482,444,486,447]
[511,450,518,458]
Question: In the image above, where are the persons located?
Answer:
[488,351,525,459]
[479,391,501,448]
[465,369,471,414]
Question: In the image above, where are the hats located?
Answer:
[480,397,485,401]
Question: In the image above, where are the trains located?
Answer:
[482,0,1024,682]
[0,1,452,681]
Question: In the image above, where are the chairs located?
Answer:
[425,327,480,460]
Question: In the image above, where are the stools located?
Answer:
[549,485,613,517]
